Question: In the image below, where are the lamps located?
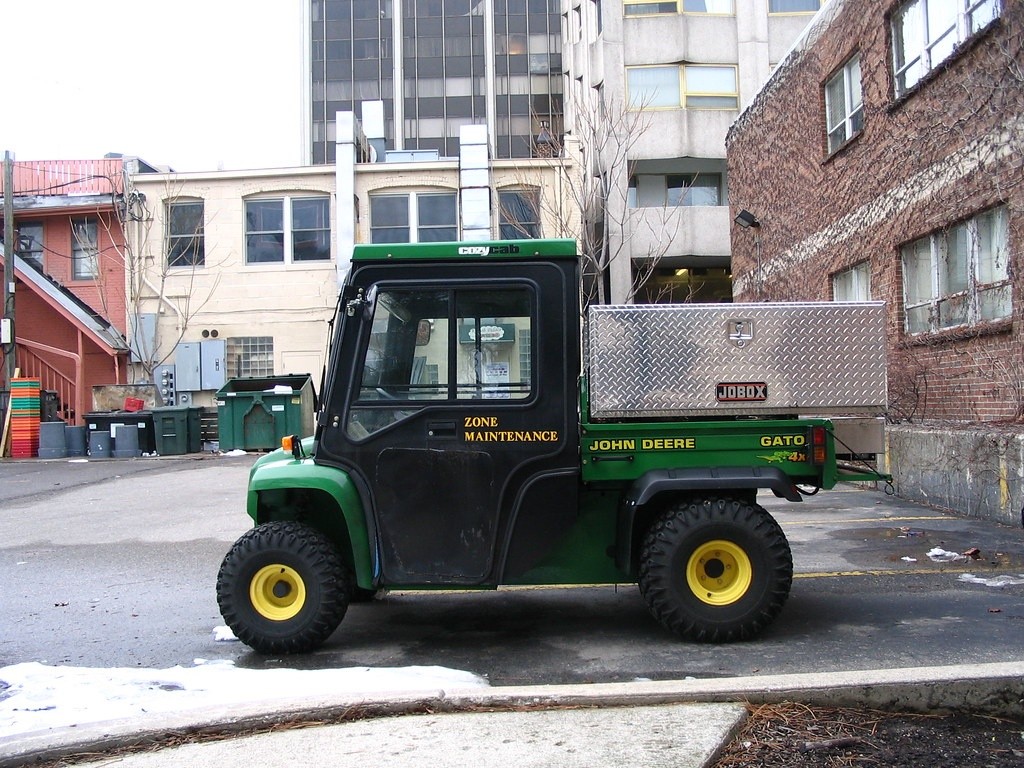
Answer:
[537,121,572,144]
[734,210,760,229]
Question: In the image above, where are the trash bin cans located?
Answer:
[38,422,142,458]
[216,372,318,453]
[188,406,205,453]
[82,408,156,456]
[39,390,58,421]
[150,405,190,455]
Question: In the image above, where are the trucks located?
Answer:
[212,238,897,653]
